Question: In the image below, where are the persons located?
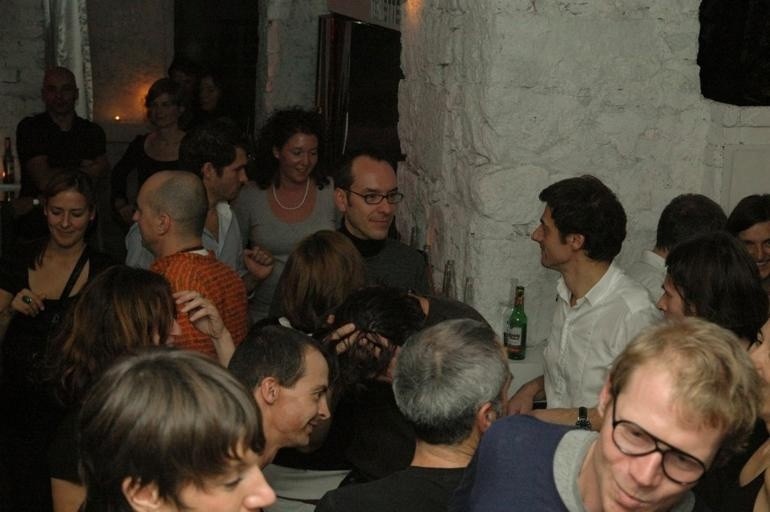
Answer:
[2,66,515,509]
[445,176,769,512]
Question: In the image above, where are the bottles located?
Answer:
[508,287,528,360]
[409,225,476,311]
[503,277,522,345]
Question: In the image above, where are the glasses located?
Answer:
[349,190,404,204]
[612,392,707,485]
